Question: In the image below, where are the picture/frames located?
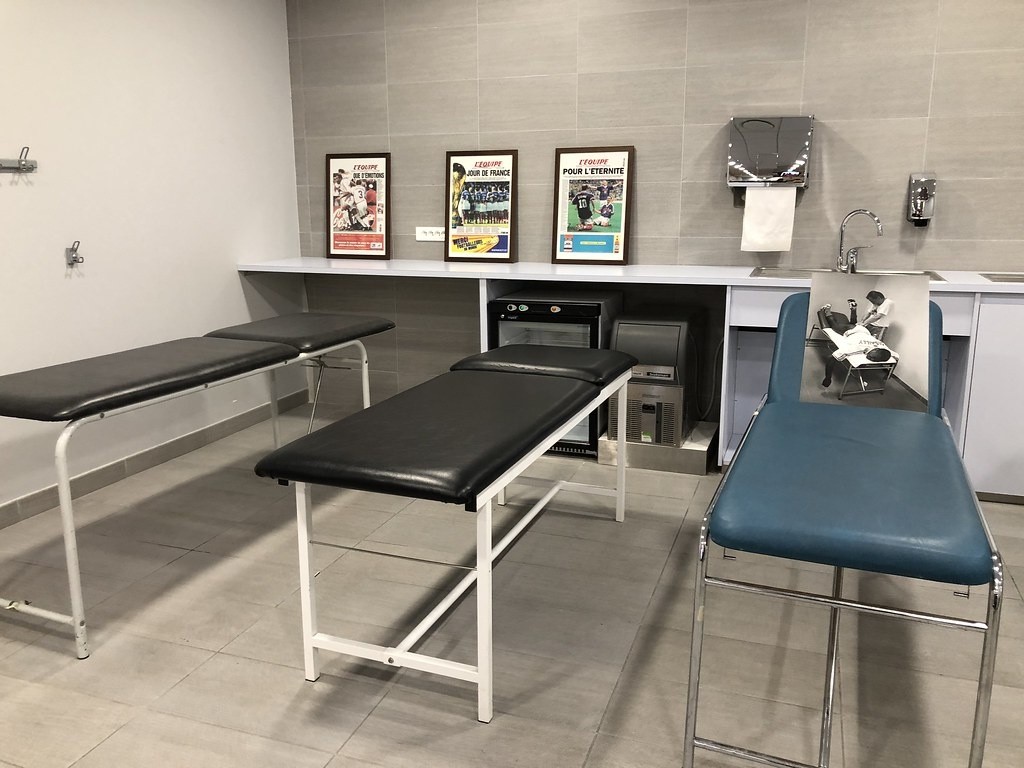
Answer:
[551,146,635,264]
[443,150,519,262]
[325,153,391,260]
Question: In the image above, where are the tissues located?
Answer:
[727,116,815,252]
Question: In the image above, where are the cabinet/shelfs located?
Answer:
[963,293,1024,498]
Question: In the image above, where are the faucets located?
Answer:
[836,209,883,272]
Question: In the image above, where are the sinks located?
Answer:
[748,266,948,281]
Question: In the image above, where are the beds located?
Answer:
[254,344,639,723]
[806,310,898,400]
[0,312,397,660]
[681,291,1006,768]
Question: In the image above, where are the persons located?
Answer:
[452,163,509,227]
[593,197,614,227]
[820,299,899,387]
[333,168,376,231]
[571,184,595,230]
[855,290,894,342]
[588,180,621,200]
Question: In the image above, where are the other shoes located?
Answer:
[367,227,372,231]
[451,223,457,228]
[847,299,858,309]
[824,303,832,317]
[464,220,509,224]
[362,226,369,231]
[574,224,584,230]
[457,223,463,226]
